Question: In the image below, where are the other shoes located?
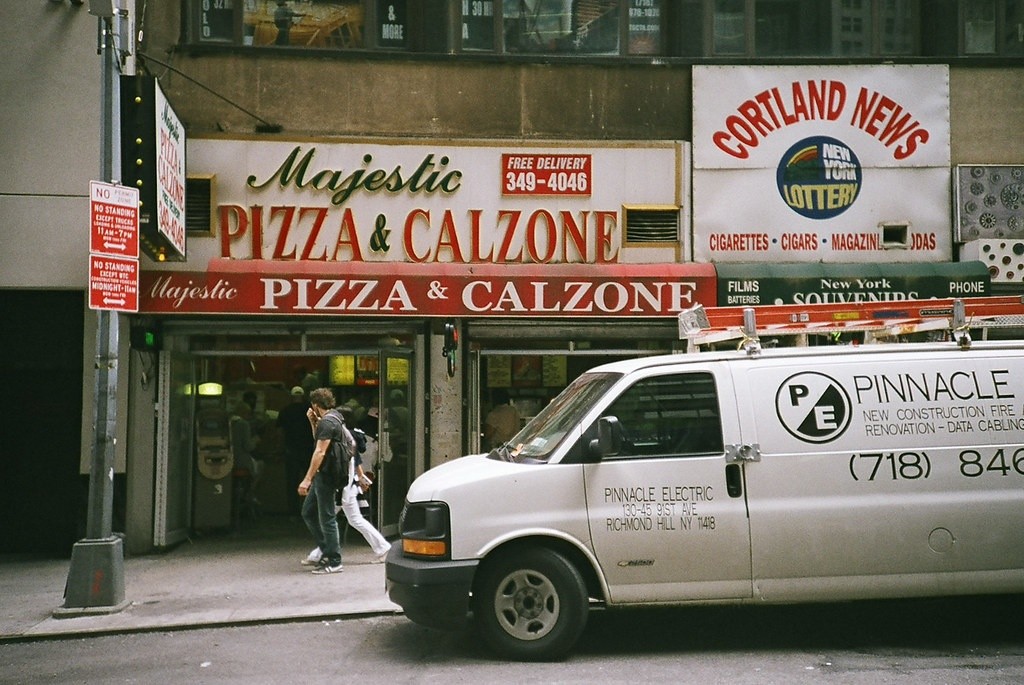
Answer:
[371,551,389,564]
[312,564,344,574]
[301,558,319,566]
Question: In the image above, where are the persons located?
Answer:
[299,388,392,574]
[232,392,265,534]
[484,388,521,453]
[384,390,409,469]
[275,386,315,535]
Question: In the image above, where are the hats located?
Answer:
[390,390,404,401]
[291,385,304,396]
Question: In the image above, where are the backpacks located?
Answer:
[326,413,357,459]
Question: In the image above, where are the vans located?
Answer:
[384,295,1024,662]
